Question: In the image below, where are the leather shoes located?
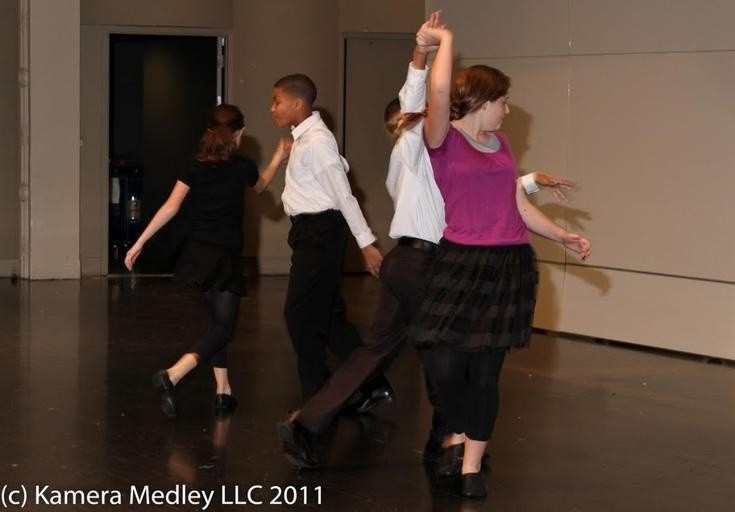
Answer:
[342,381,397,418]
[461,468,487,498]
[423,439,493,479]
[212,393,238,411]
[155,369,179,415]
[276,411,321,472]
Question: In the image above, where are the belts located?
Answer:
[287,210,344,226]
[396,236,440,254]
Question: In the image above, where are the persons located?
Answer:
[415,18,590,500]
[122,102,291,419]
[274,9,582,471]
[270,73,396,439]
